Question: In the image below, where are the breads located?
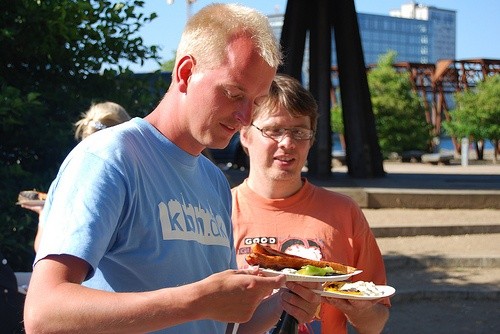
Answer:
[244,243,347,273]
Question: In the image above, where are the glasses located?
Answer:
[250,123,316,140]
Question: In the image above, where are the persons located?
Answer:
[17,101,132,255]
[227,75,391,334]
[23,4,321,334]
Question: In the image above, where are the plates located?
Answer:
[311,285,396,300]
[248,265,362,282]
